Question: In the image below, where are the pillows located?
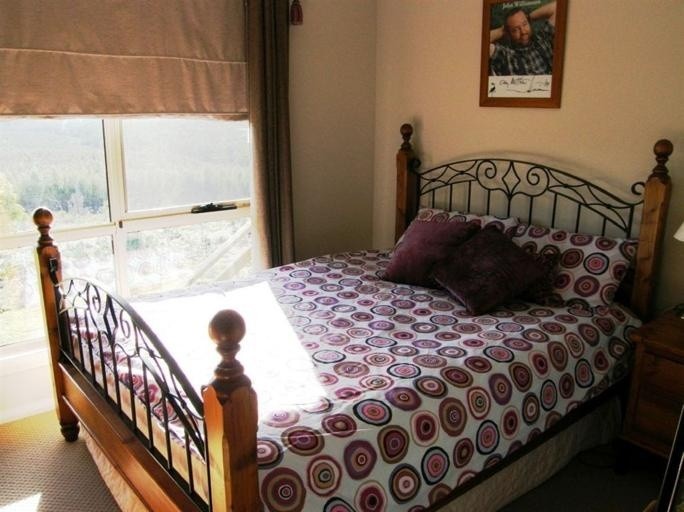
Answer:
[378,206,638,315]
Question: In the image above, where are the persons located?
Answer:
[486,1,555,75]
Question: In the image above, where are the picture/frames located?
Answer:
[478,0,567,109]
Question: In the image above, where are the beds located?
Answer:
[29,122,680,511]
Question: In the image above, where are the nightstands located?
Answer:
[619,345,684,468]
